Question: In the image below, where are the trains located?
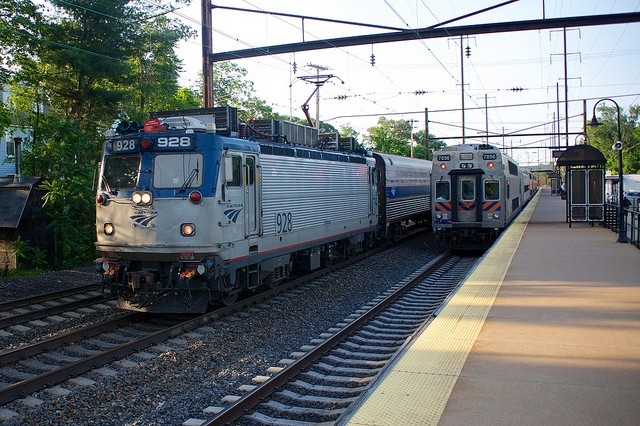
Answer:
[94,107,432,313]
[431,144,540,251]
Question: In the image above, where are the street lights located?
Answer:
[587,98,628,243]
